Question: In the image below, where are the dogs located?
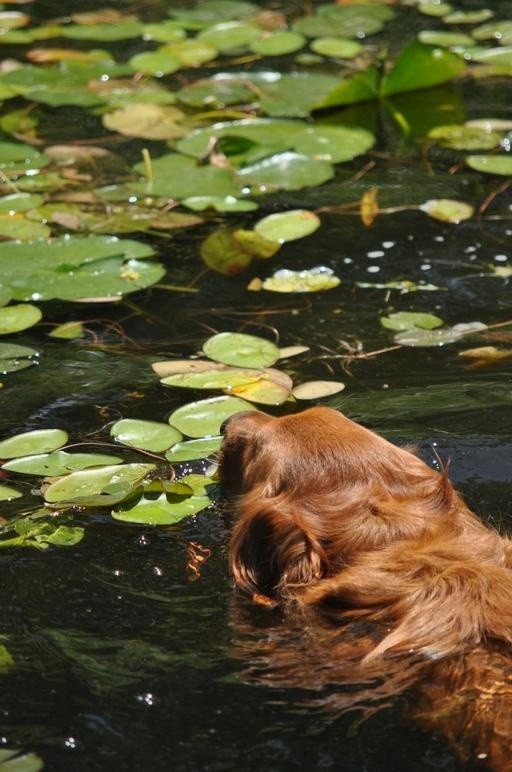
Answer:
[214,403,512,772]
